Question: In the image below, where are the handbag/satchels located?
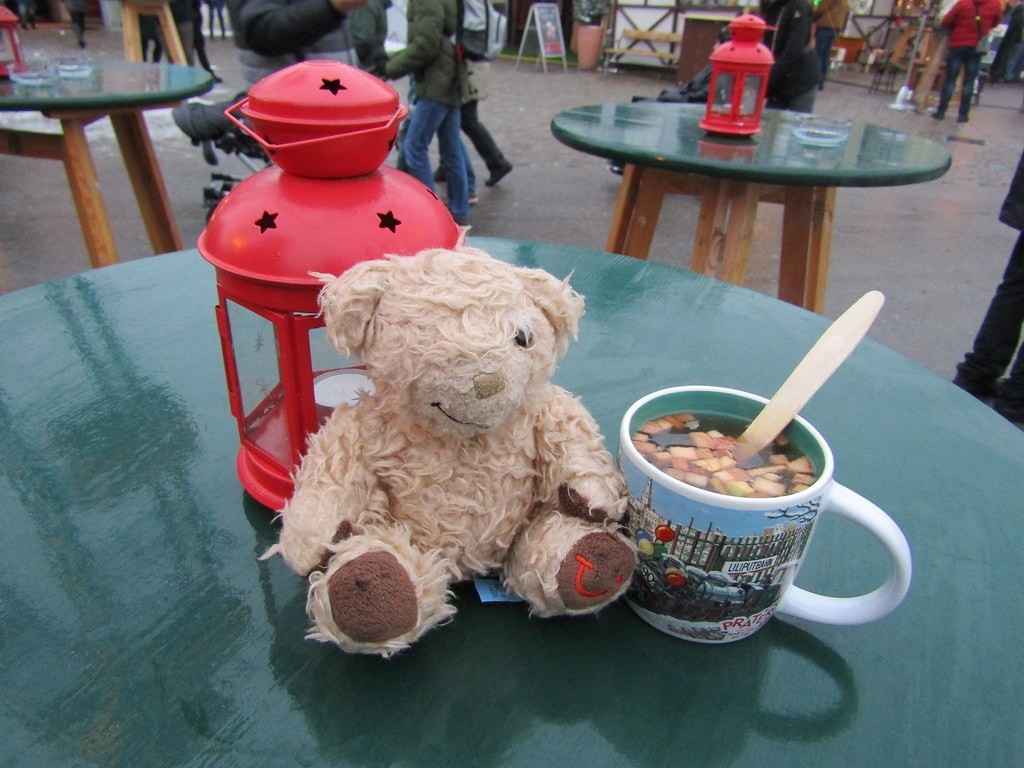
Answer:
[975,36,990,55]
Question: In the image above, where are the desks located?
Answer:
[0,59,213,296]
[553,103,952,316]
[0,236,1024,768]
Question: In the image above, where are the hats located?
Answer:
[719,26,731,43]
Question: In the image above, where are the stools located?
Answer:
[869,63,898,95]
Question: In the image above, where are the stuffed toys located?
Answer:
[273,230,640,659]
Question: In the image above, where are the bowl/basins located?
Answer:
[791,114,854,144]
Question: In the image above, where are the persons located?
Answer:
[138,0,225,84]
[931,0,1003,122]
[225,0,371,87]
[953,149,1024,429]
[376,0,513,230]
[811,0,847,89]
[66,0,87,47]
[18,0,40,30]
[758,0,820,114]
[680,25,734,104]
[348,0,394,73]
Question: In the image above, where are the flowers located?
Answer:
[574,0,607,26]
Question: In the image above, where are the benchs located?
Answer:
[605,31,682,74]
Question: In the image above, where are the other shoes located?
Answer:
[431,164,446,184]
[485,161,512,187]
[952,371,1001,397]
[207,70,224,84]
[453,216,473,231]
[992,393,1024,422]
[440,190,479,206]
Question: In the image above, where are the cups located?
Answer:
[619,386,912,645]
[7,30,59,86]
[50,26,94,78]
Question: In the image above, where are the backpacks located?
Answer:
[456,0,508,62]
[766,0,825,106]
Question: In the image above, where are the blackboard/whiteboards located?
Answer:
[518,3,566,58]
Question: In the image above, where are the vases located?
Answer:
[577,26,603,70]
